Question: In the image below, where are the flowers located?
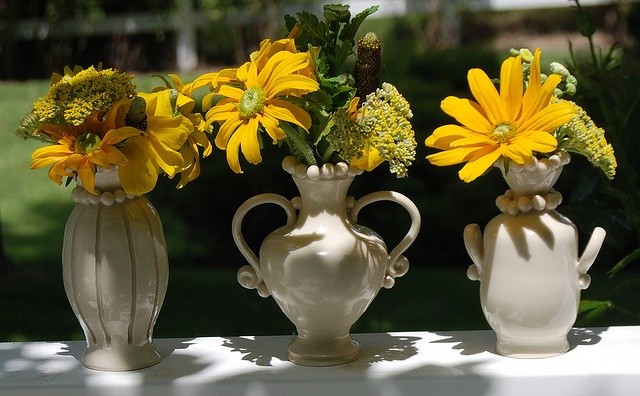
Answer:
[14,61,216,196]
[424,47,619,183]
[181,4,417,180]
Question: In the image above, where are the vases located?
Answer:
[61,164,169,373]
[463,153,608,359]
[231,155,422,368]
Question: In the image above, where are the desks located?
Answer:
[1,327,640,396]
